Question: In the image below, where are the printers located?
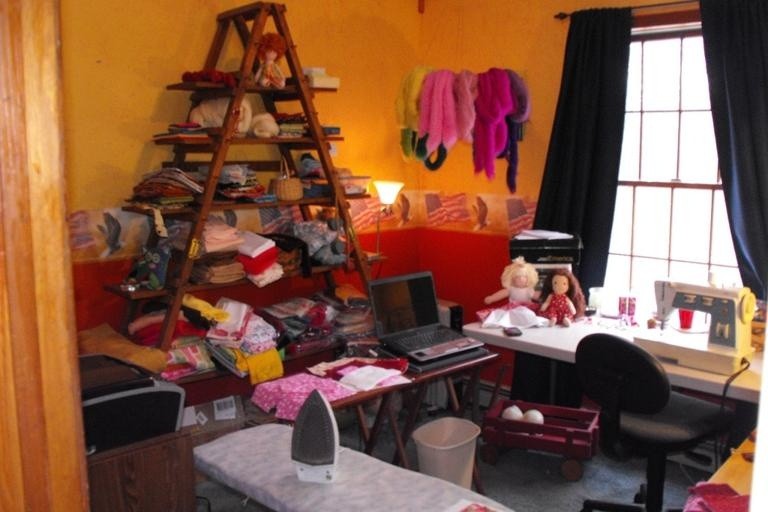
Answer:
[79,353,186,455]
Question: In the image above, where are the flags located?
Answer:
[424,193,471,225]
[349,199,395,232]
[68,210,96,250]
[506,199,538,238]
[260,205,304,237]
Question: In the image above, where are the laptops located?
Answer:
[367,271,486,363]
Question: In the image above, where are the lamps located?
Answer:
[370,180,405,264]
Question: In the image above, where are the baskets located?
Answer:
[269,155,304,201]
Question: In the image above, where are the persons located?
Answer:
[481,255,550,330]
[539,268,586,328]
[398,194,411,224]
[96,212,122,252]
[472,196,488,230]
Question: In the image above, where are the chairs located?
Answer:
[575,332,740,512]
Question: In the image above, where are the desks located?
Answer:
[191,422,518,511]
[463,302,763,464]
[253,344,500,498]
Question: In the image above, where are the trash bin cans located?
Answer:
[411,417,481,490]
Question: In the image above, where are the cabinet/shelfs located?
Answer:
[101,2,390,387]
